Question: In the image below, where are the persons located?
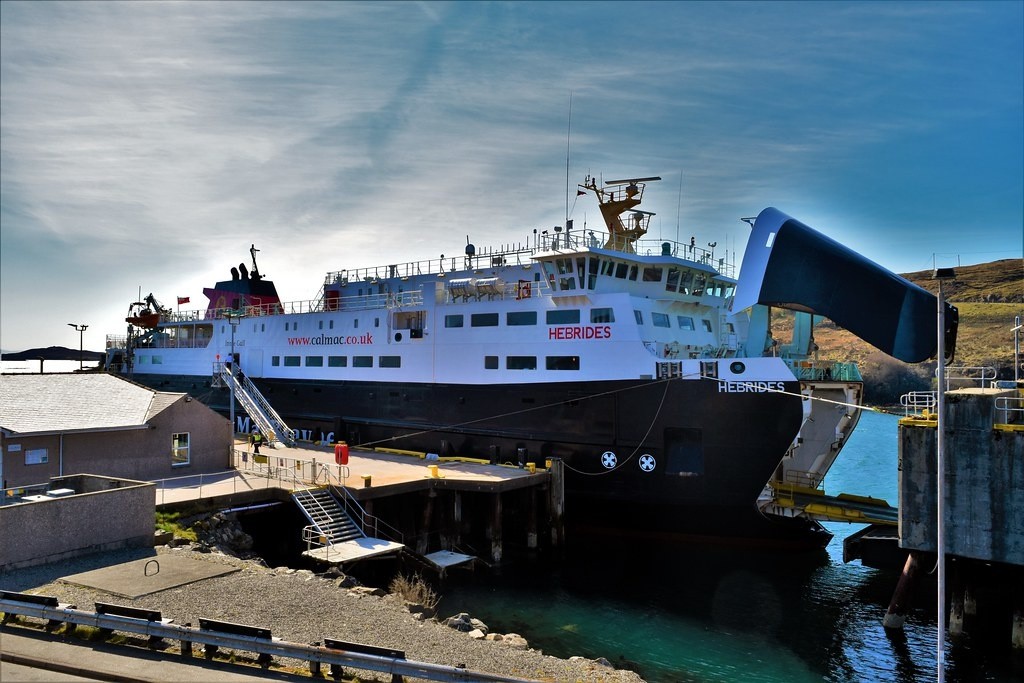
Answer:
[250,431,262,458]
[237,368,245,387]
[224,352,234,374]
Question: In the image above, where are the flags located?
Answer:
[178,296,190,305]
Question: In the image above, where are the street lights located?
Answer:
[68,323,89,372]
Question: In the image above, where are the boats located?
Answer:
[103,174,867,551]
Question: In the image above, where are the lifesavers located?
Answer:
[520,285,531,298]
